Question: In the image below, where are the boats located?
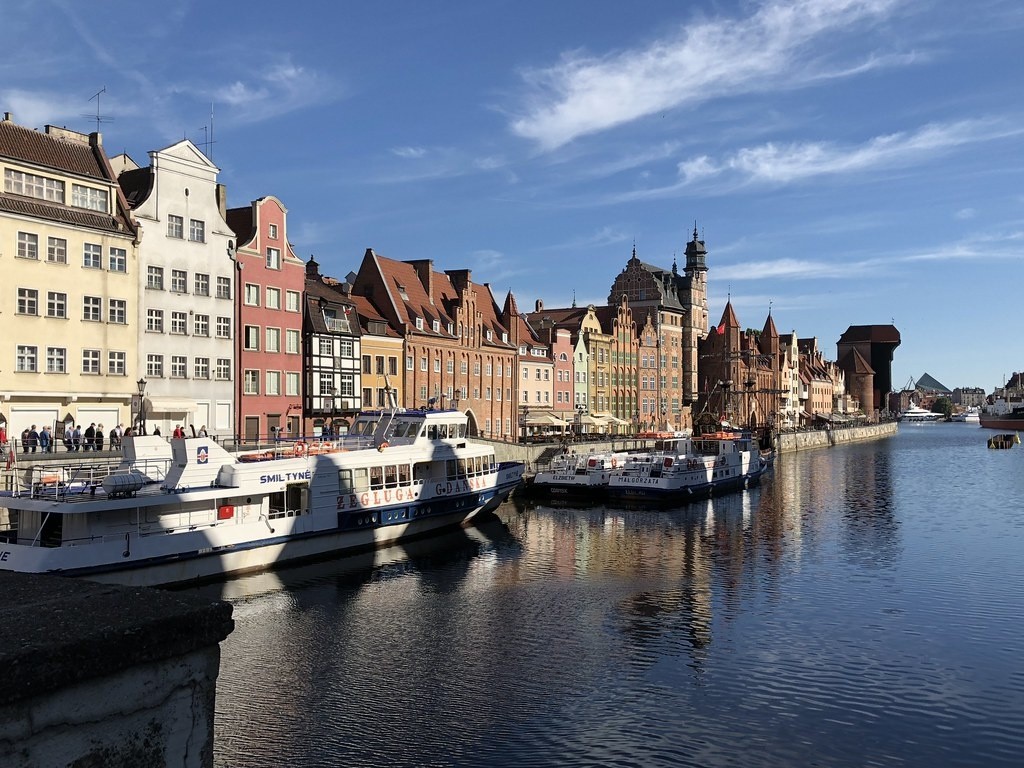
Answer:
[532,327,778,504]
[898,394,944,422]
[987,434,1016,449]
[949,411,984,421]
[0,375,530,593]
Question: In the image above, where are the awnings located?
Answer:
[788,411,867,422]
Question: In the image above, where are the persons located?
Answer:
[63,423,138,451]
[570,429,575,442]
[433,425,453,439]
[0,427,8,453]
[153,426,161,436]
[173,424,208,438]
[21,425,54,454]
[321,423,336,440]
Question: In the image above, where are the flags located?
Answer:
[717,323,726,334]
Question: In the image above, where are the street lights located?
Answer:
[137,377,148,436]
[456,388,461,411]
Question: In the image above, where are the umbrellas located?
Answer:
[526,415,569,436]
[577,415,630,433]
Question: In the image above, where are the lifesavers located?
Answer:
[611,458,617,468]
[293,441,332,456]
[688,459,697,469]
